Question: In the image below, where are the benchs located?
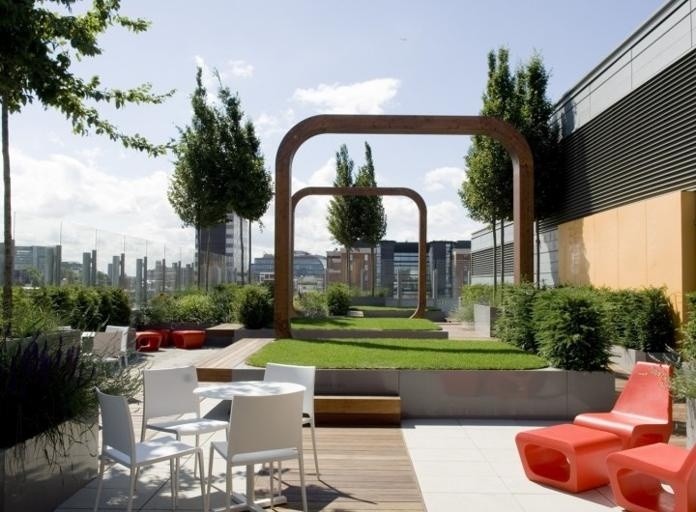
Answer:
[205,322,245,343]
[194,338,274,380]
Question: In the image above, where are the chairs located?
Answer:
[56,324,130,381]
[574,359,695,512]
[91,359,326,512]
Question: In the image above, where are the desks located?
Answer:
[134,330,161,352]
[512,423,618,498]
[172,329,206,350]
